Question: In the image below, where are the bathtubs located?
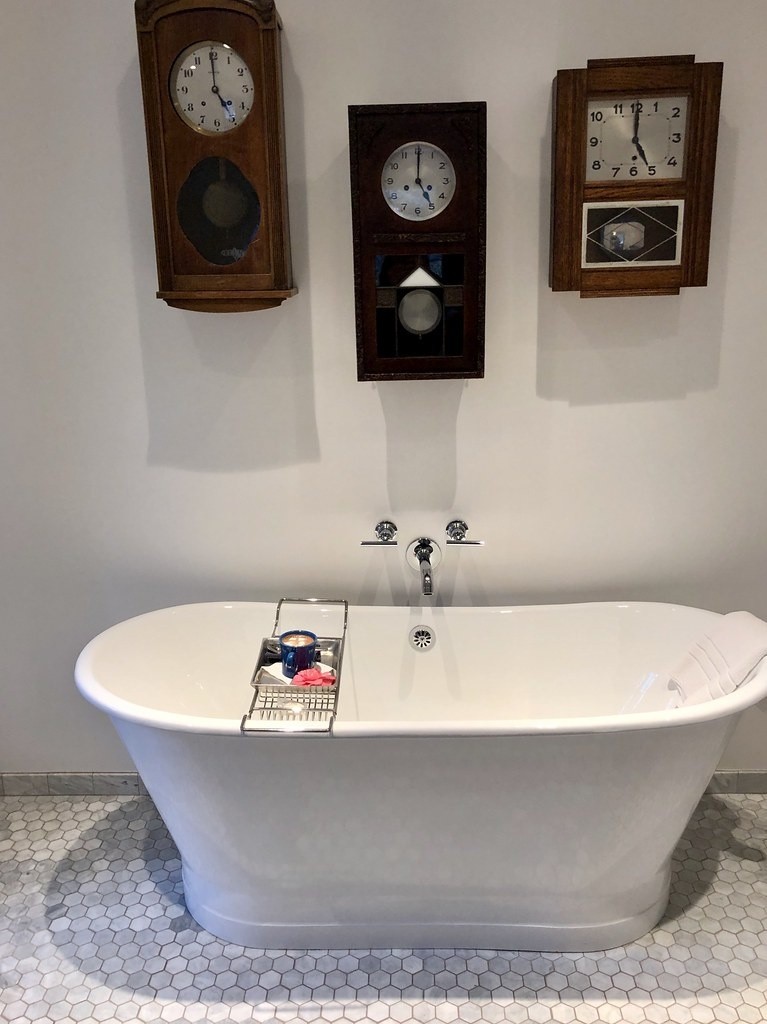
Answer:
[73,601,767,952]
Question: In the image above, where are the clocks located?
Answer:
[548,55,724,298]
[348,100,487,382]
[134,0,299,313]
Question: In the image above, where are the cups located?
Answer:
[278,629,317,679]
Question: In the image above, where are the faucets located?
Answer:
[405,537,442,596]
